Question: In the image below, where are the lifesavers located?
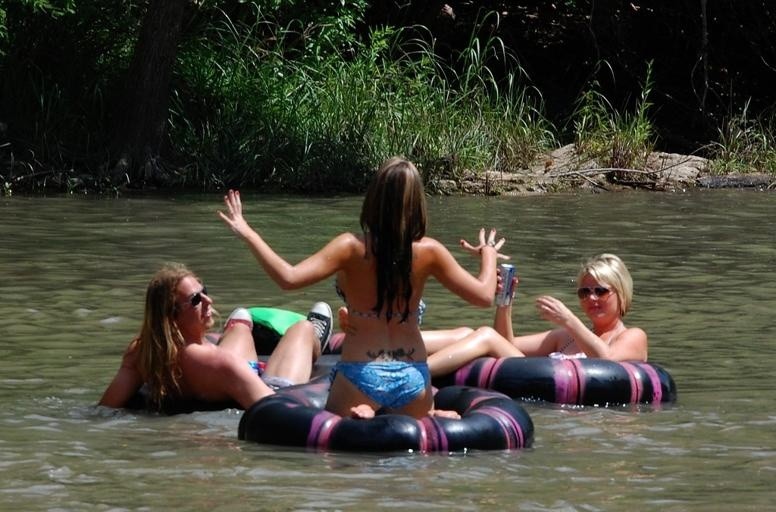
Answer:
[126,332,676,452]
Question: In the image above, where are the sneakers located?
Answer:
[307,300,334,355]
[222,306,254,331]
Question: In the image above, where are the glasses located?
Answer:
[177,285,208,307]
[577,286,614,299]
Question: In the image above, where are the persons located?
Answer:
[216,158,511,419]
[99,261,334,411]
[338,253,648,379]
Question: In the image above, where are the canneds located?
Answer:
[494,264,515,307]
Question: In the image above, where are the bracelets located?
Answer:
[479,240,498,254]
